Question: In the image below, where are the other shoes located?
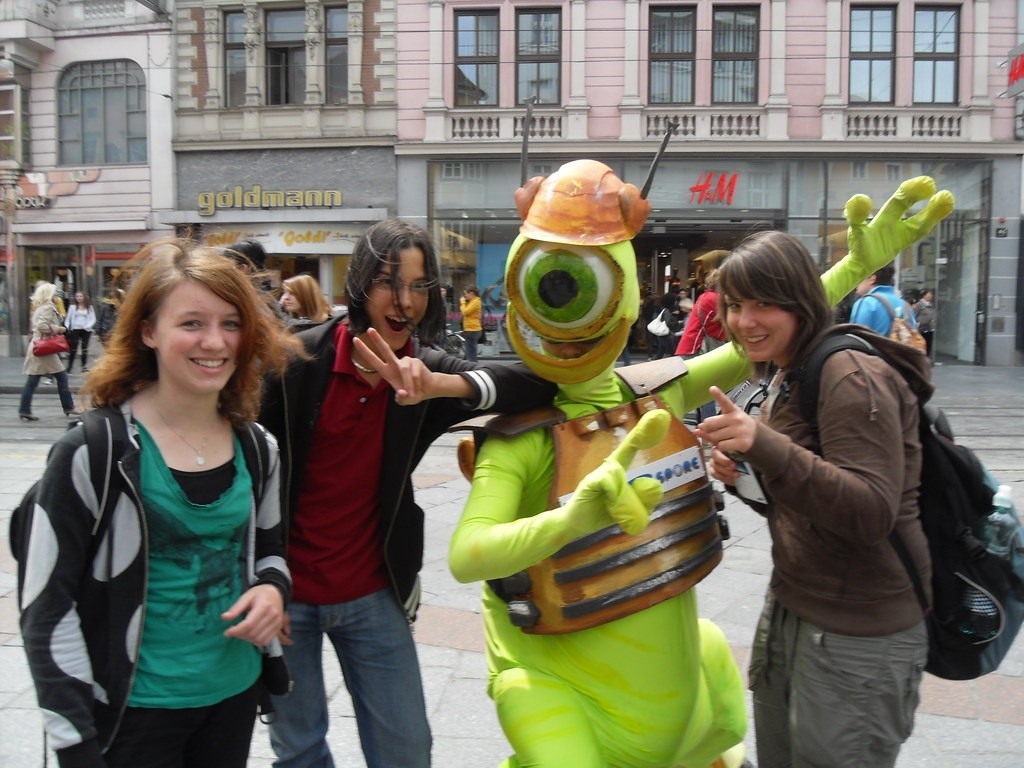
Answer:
[81,366,88,371]
[45,378,53,384]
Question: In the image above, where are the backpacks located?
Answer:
[801,334,1024,681]
[860,292,927,356]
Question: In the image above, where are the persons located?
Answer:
[253,218,562,768]
[849,264,938,360]
[621,285,696,365]
[19,224,319,768]
[438,285,482,362]
[18,279,127,423]
[693,229,928,768]
[448,162,953,768]
[224,239,335,336]
[673,271,732,358]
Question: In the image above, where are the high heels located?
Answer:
[19,412,40,421]
[63,406,85,416]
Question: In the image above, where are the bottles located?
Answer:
[960,484,1019,637]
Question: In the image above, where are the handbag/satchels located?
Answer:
[32,325,70,357]
[699,336,725,356]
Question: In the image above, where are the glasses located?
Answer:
[368,273,430,299]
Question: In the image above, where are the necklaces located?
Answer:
[143,394,219,466]
[350,358,380,374]
